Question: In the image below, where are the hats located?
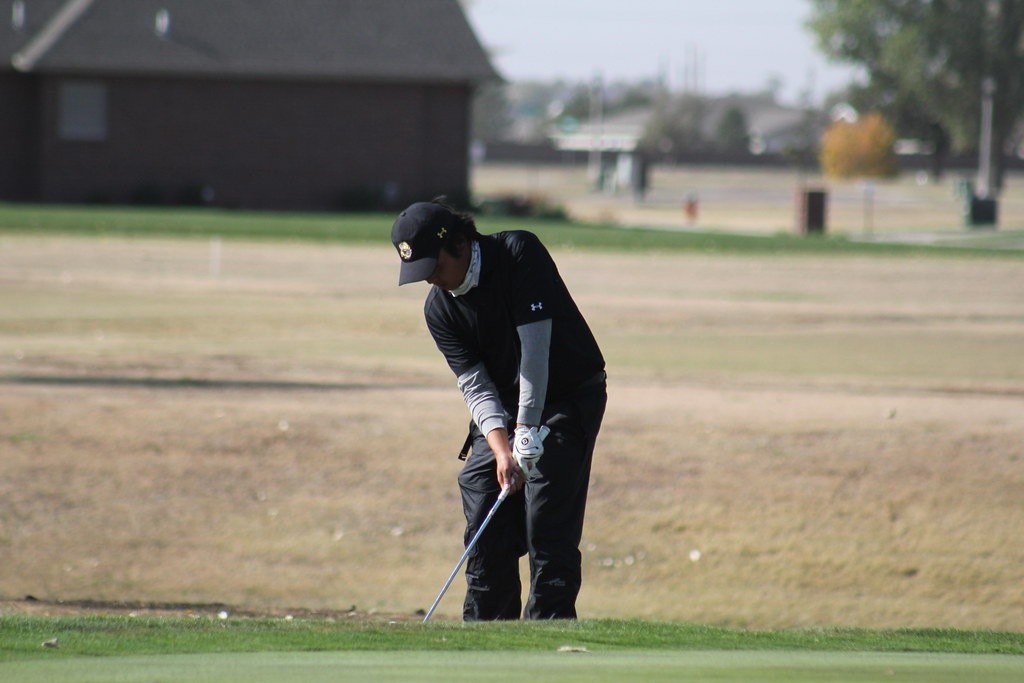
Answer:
[390,200,453,286]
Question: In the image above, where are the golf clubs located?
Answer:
[422,424,550,622]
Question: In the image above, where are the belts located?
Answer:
[547,368,607,403]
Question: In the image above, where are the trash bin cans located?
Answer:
[808,191,824,231]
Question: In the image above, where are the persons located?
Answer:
[391,202,608,620]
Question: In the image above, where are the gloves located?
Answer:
[511,424,545,481]
